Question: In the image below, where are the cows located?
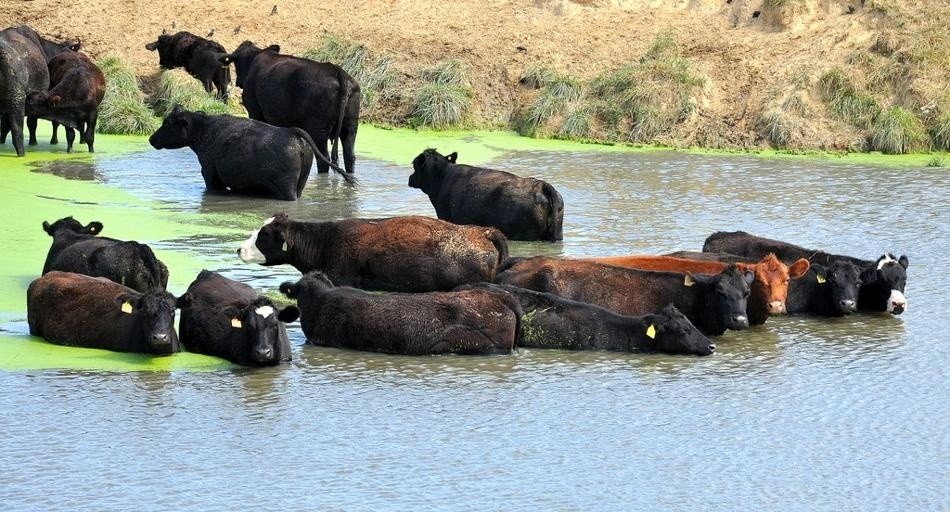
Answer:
[27,208,909,357]
[407,146,563,242]
[1,23,361,204]
